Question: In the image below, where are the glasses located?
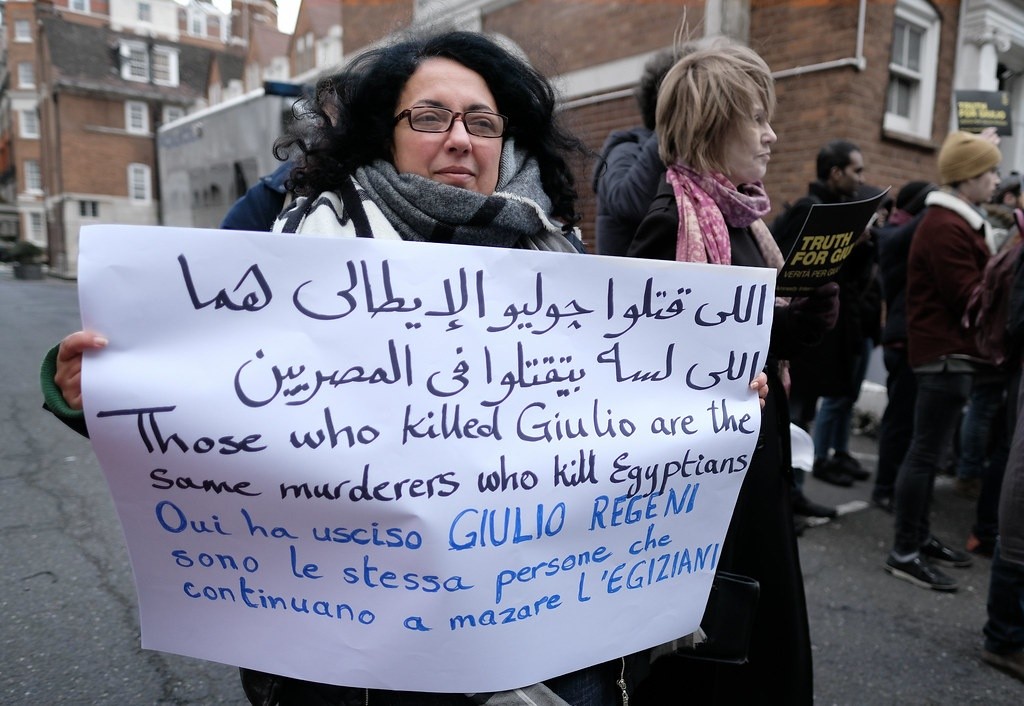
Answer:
[393,106,507,139]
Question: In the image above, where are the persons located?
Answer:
[768,128,1024,681]
[45,28,768,706]
[606,50,840,706]
[592,40,699,256]
[214,68,340,232]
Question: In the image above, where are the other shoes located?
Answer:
[966,534,998,556]
[798,490,840,526]
[814,456,869,485]
[951,477,981,499]
[872,489,895,512]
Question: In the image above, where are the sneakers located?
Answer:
[883,553,961,593]
[983,639,1024,678]
[921,535,973,567]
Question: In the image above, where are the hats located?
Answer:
[937,130,999,184]
[996,172,1021,197]
